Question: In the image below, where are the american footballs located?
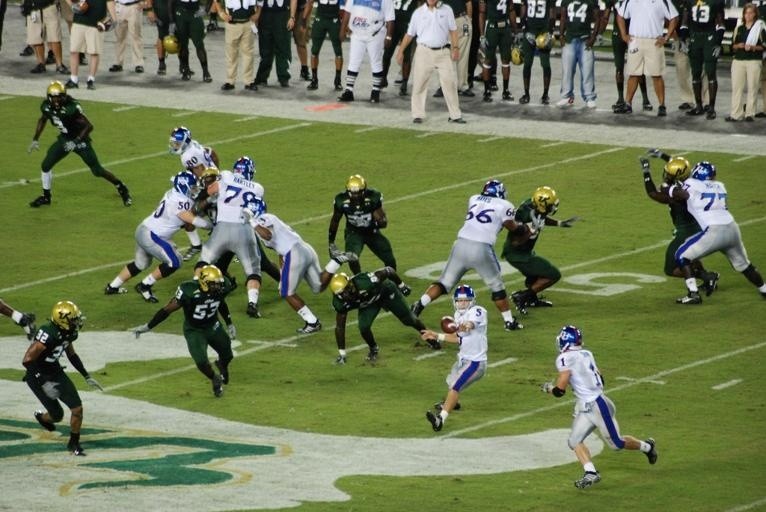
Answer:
[442,316,457,334]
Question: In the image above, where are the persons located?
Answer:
[331,266,442,364]
[197,155,264,318]
[502,185,579,315]
[0,0,7,50]
[519,0,557,104]
[211,0,265,91]
[336,0,396,103]
[473,33,499,92]
[613,1,680,118]
[418,286,490,430]
[253,0,297,86]
[328,173,398,274]
[20,6,59,64]
[152,1,196,75]
[126,264,238,398]
[743,41,766,118]
[169,0,213,82]
[23,0,71,75]
[541,326,658,488]
[379,0,422,95]
[105,167,213,306]
[600,0,653,112]
[674,159,766,303]
[726,2,766,122]
[302,0,343,91]
[479,0,518,102]
[192,169,283,295]
[637,148,720,299]
[28,81,135,207]
[59,1,89,66]
[558,0,602,108]
[168,127,220,258]
[0,295,38,342]
[65,0,118,89]
[288,0,312,82]
[678,0,725,121]
[395,0,468,125]
[23,299,106,458]
[241,203,359,334]
[108,0,150,73]
[673,0,716,111]
[412,179,524,332]
[432,0,476,98]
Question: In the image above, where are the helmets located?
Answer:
[200,169,219,177]
[481,178,506,200]
[241,198,266,223]
[199,264,224,297]
[531,186,560,216]
[51,300,86,333]
[47,82,67,111]
[328,272,357,303]
[663,156,691,183]
[511,48,523,66]
[452,284,477,314]
[536,32,553,51]
[169,127,192,155]
[691,160,716,181]
[555,324,584,351]
[345,174,366,202]
[170,172,201,201]
[233,155,257,181]
[162,35,181,54]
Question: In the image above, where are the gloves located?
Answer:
[98,16,114,32]
[513,34,520,47]
[335,355,347,368]
[400,284,412,296]
[529,209,546,232]
[711,45,722,59]
[42,381,62,400]
[539,377,556,393]
[127,323,150,334]
[244,208,260,229]
[645,148,663,159]
[679,37,691,54]
[560,215,578,229]
[524,31,536,47]
[70,0,88,14]
[62,141,77,152]
[639,157,650,173]
[329,243,338,256]
[354,218,369,228]
[480,35,489,50]
[27,140,40,154]
[227,324,236,340]
[86,378,105,393]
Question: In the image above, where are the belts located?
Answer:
[228,18,250,24]
[114,1,142,8]
[422,42,450,51]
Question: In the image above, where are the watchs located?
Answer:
[452,44,461,47]
[663,36,668,41]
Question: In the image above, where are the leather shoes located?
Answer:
[434,88,443,97]
[448,117,467,124]
[724,117,739,122]
[473,76,484,81]
[679,103,693,109]
[704,104,711,110]
[746,117,753,121]
[463,90,475,97]
[491,85,498,91]
[244,84,258,91]
[468,83,473,88]
[414,118,422,124]
[755,113,766,118]
[221,83,235,90]
[394,79,403,83]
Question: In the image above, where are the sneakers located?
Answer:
[31,195,51,208]
[542,95,549,104]
[105,283,128,295]
[587,101,597,108]
[133,281,159,304]
[519,96,530,103]
[434,400,461,410]
[643,103,652,110]
[332,249,359,263]
[281,81,289,87]
[203,74,212,82]
[365,345,380,362]
[307,81,318,89]
[430,342,441,351]
[56,64,71,75]
[183,74,190,80]
[381,80,388,88]
[214,358,229,385]
[182,245,203,261]
[34,410,56,432]
[212,372,225,398]
[556,98,574,107]
[109,65,122,72]
[658,105,667,116]
[86,80,95,90]
[707,111,716,119]
[686,108,705,117]
[425,409,444,432]
[18,312,38,341]
[697,283,707,292]
[229,276,237,290]
[676,290,702,305]
[504,317,524,331]
[245,302,262,319]
[574,470,603,489]
[64,81,79,89]
[370,90,380,102]
[119,184,133,208]
[295,319,323,335]
[255,81,268,88]
[612,102,625,109]
[45,57,57,64]
[158,67,167,74]
[334,79,343,90]
[704,271,721,297]
[81,58,88,64]
[68,443,84,456]
[30,64,46,74]
[643,438,658,465]
[510,291,529,316]
[410,299,423,316]
[20,46,34,57]
[337,90,354,101]
[300,69,312,81]
[527,298,553,307]
[614,105,633,114]
[136,66,144,73]
[179,67,195,75]
[502,91,514,100]
[484,92,493,103]
[399,89,407,95]
